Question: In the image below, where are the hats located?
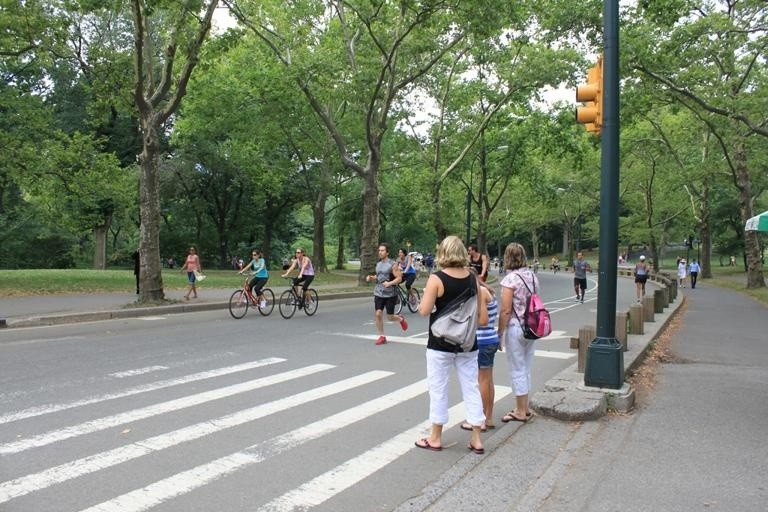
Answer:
[679,258,687,263]
[640,255,646,260]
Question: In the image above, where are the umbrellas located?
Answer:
[745,210,767,232]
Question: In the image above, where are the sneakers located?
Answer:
[375,336,387,345]
[400,316,409,332]
[679,283,696,289]
[307,300,314,311]
[575,294,585,303]
[260,298,268,309]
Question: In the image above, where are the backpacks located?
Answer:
[511,272,553,340]
[430,272,480,353]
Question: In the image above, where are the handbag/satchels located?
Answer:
[193,269,208,283]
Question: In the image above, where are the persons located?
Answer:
[180,246,202,299]
[393,248,420,306]
[232,255,238,270]
[132,247,141,294]
[618,250,628,267]
[365,242,409,344]
[166,256,173,268]
[413,237,551,454]
[676,254,701,288]
[571,252,591,303]
[237,249,269,309]
[730,255,736,267]
[290,256,297,266]
[283,255,289,270]
[281,248,315,305]
[634,255,650,304]
[552,256,559,271]
[238,257,243,270]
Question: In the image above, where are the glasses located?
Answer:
[252,253,258,256]
[468,249,473,252]
[295,251,303,254]
[189,249,196,251]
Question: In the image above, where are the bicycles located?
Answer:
[394,283,421,316]
[229,272,275,319]
[278,276,319,319]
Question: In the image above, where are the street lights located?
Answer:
[557,187,581,255]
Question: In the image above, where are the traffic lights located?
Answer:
[574,58,603,133]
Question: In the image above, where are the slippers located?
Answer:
[468,442,485,455]
[414,436,443,451]
[460,422,496,434]
[501,409,532,423]
